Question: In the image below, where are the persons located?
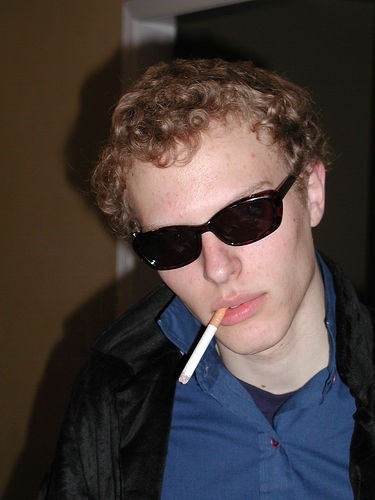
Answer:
[33,59,375,500]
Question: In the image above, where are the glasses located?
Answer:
[130,161,304,270]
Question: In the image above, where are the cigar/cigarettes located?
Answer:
[179,307,226,384]
